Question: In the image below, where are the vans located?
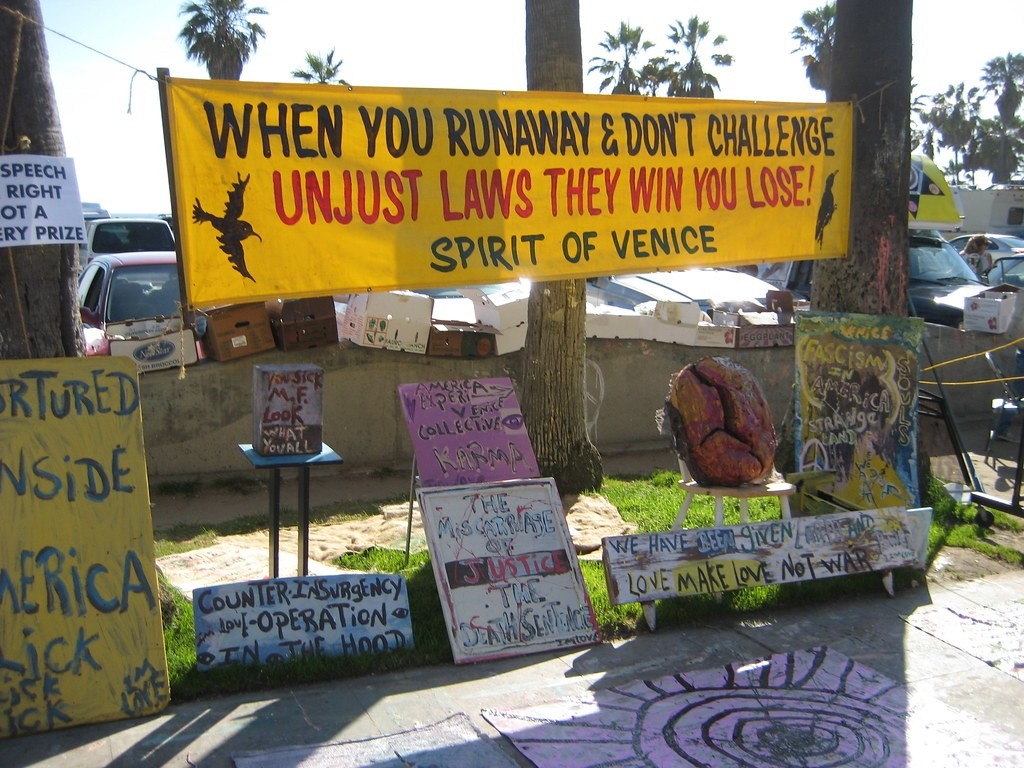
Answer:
[76,216,178,282]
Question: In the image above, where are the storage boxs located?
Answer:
[964,283,1022,334]
[711,291,795,348]
[271,295,339,353]
[344,290,435,355]
[195,300,275,362]
[105,315,200,374]
[458,277,528,355]
[427,318,503,357]
[583,298,739,348]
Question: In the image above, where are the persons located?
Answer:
[959,235,993,282]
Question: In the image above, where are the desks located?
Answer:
[237,441,343,578]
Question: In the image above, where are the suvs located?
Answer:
[757,227,992,329]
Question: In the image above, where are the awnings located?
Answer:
[908,155,963,232]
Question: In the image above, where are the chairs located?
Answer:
[673,436,798,532]
[983,351,1024,471]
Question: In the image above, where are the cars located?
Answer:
[329,277,529,343]
[74,252,205,361]
[948,233,1024,290]
[581,268,795,327]
